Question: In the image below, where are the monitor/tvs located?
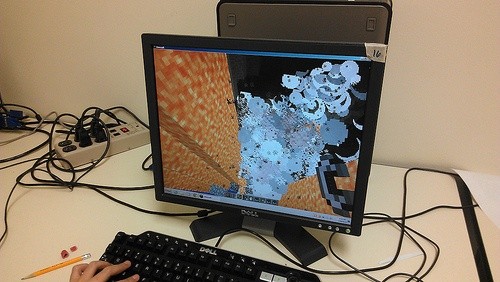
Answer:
[142,31,390,237]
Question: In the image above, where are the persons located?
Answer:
[69,261,140,282]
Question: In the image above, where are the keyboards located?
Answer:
[94,232,318,282]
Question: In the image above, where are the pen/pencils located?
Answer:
[21,252,91,280]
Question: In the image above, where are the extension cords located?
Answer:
[53,119,150,170]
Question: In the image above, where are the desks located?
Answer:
[0,116,500,281]
